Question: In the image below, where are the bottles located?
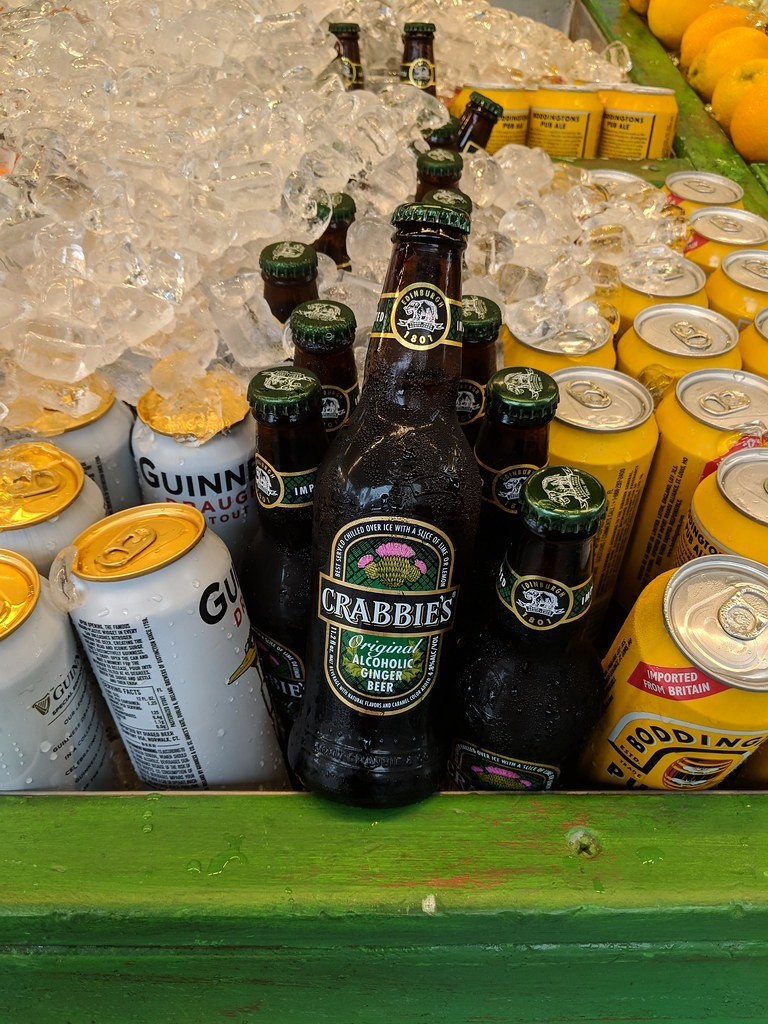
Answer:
[234,366,328,734]
[401,23,435,96]
[458,295,502,451]
[474,366,560,581]
[288,202,481,808]
[423,91,502,152]
[290,298,362,453]
[414,148,462,203]
[314,193,356,271]
[422,188,472,271]
[328,23,363,92]
[259,242,320,324]
[441,467,607,792]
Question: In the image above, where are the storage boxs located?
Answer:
[0,1,767,1022]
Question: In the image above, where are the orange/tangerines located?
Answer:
[623,0,768,164]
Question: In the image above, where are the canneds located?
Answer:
[435,74,679,164]
[482,168,768,788]
[0,355,299,794]
